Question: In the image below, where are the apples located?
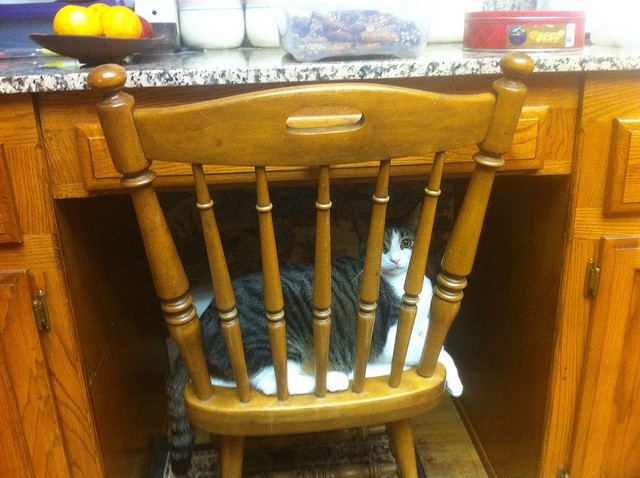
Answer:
[137,15,153,37]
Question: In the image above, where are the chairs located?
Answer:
[86,54,534,478]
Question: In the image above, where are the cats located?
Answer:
[162,200,465,476]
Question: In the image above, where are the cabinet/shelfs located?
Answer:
[527,71,640,477]
[0,92,133,477]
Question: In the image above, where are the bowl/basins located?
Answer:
[28,32,150,70]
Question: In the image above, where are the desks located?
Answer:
[0,51,640,203]
